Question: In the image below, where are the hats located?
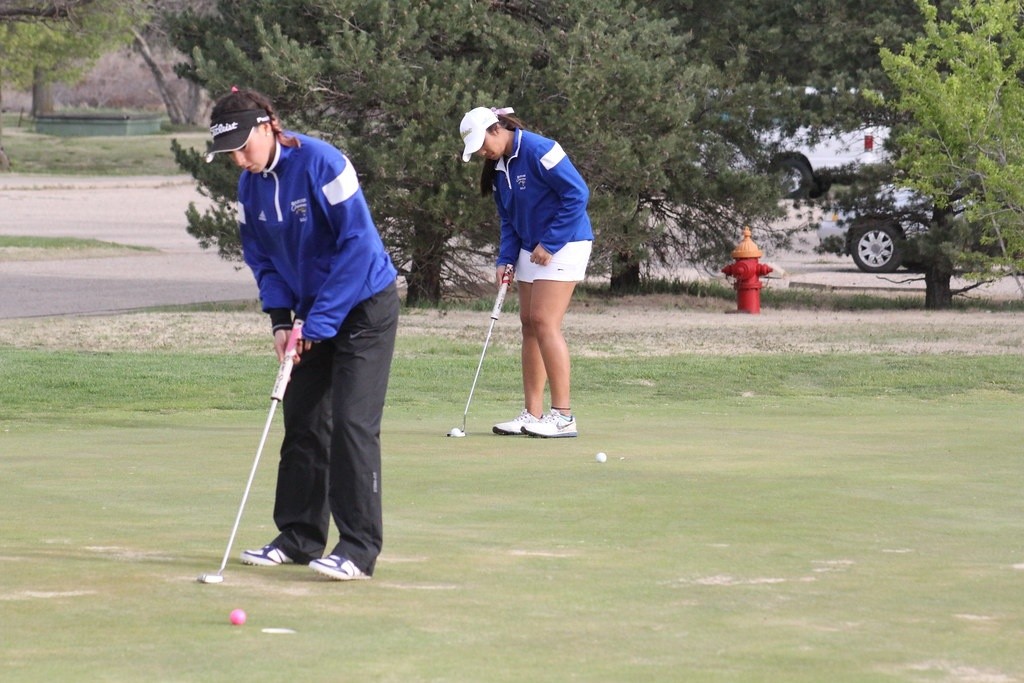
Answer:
[460,107,500,162]
[203,109,269,163]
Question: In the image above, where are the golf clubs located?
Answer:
[450,264,512,437]
[198,307,305,584]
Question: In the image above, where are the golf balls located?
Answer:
[596,452,608,464]
[230,609,247,625]
[451,428,461,438]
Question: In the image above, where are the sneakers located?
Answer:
[493,409,546,435]
[308,554,372,580]
[520,409,578,437]
[239,544,294,567]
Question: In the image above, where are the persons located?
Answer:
[206,87,399,580]
[459,107,594,438]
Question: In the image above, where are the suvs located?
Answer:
[686,82,900,198]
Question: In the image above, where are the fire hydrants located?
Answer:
[721,225,774,314]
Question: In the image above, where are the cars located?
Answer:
[815,166,978,274]
